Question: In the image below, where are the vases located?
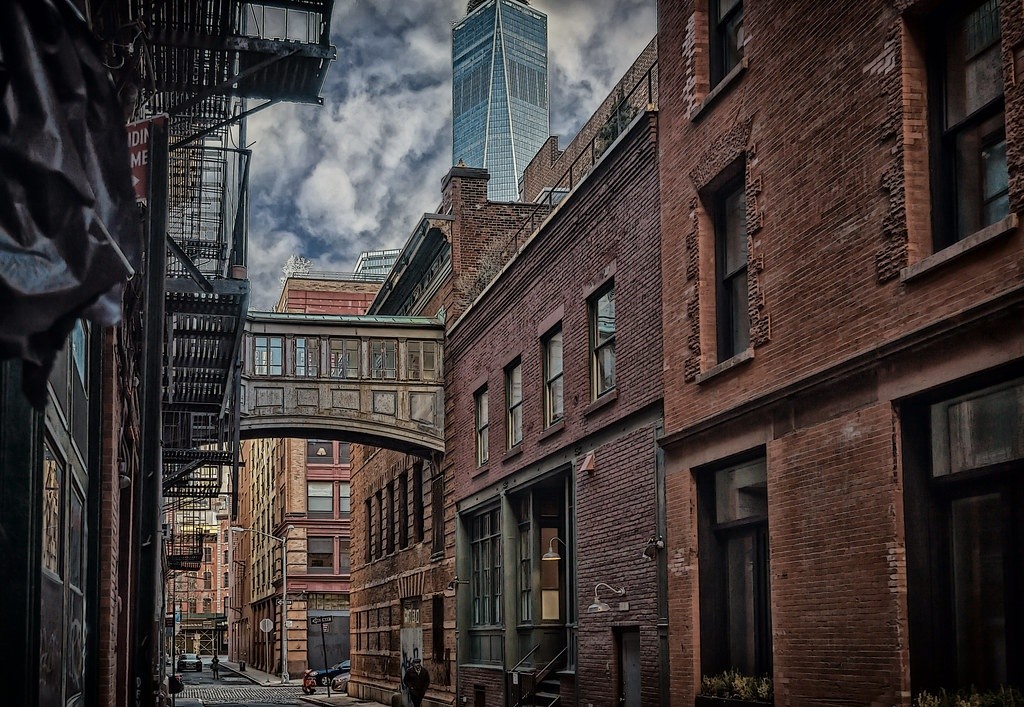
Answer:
[695,695,773,707]
[231,265,247,280]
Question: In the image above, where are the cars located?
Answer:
[315,658,350,687]
[177,653,202,673]
[331,672,350,693]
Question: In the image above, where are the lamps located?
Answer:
[586,583,627,614]
[542,536,566,560]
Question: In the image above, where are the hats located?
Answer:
[413,659,421,662]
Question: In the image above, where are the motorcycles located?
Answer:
[301,669,317,695]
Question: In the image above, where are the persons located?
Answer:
[175,648,183,657]
[211,655,220,680]
[403,659,430,707]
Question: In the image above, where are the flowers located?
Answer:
[699,668,774,703]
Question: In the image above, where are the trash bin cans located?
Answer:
[240,661,245,671]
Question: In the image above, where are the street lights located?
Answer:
[231,523,295,684]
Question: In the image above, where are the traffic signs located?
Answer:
[276,598,293,606]
[310,615,333,624]
